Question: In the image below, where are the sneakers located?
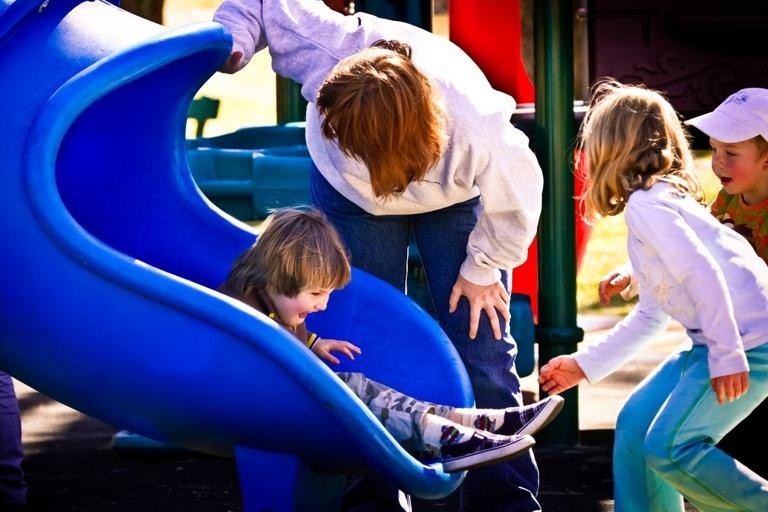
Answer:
[439,425,536,473]
[491,392,566,437]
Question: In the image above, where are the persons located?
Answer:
[596,87,768,482]
[214,201,565,473]
[210,1,545,512]
[537,76,767,512]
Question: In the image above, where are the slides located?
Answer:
[1,0,476,500]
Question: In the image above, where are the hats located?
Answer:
[682,87,768,146]
